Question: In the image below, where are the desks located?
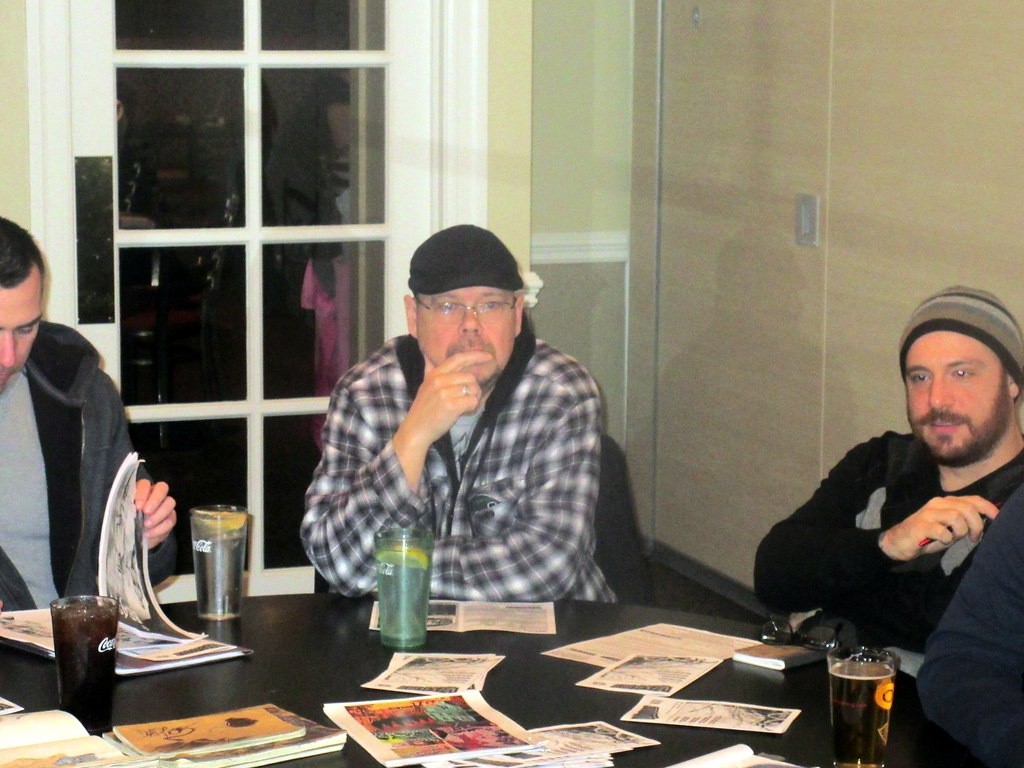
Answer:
[0,593,971,768]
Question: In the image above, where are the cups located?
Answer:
[826,646,901,768]
[50,596,119,683]
[190,505,248,621]
[375,528,434,648]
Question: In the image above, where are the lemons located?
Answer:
[376,547,429,571]
[193,510,247,532]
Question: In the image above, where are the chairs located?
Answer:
[315,436,659,604]
[119,174,239,494]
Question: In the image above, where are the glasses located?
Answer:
[414,296,517,317]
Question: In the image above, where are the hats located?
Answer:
[407,223,525,296]
[900,285,1024,403]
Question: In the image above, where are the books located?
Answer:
[0,452,259,674]
[732,641,830,673]
[0,689,802,768]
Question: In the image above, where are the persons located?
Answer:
[300,223,617,604]
[1,216,178,610]
[752,284,1024,768]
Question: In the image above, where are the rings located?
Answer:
[462,385,470,397]
